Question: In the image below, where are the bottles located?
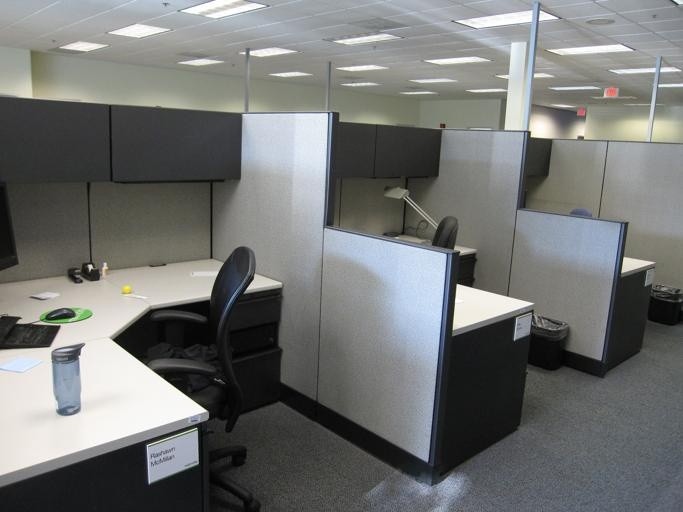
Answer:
[49,341,86,416]
[100,261,108,277]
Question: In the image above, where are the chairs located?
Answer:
[131,244,261,511]
[430,213,457,249]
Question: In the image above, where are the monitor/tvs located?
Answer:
[0,182,19,271]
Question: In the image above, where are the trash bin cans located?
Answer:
[528,315,569,370]
[648,285,683,325]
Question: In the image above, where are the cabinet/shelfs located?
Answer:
[459,250,477,284]
[221,286,282,418]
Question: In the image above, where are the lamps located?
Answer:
[382,185,441,232]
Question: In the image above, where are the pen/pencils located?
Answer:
[149,264,166,267]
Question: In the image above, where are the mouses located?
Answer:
[45,308,75,320]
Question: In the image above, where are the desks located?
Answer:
[1,258,282,492]
[377,235,537,478]
[604,253,657,372]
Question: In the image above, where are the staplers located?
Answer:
[68,266,83,283]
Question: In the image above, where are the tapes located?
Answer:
[87,263,93,272]
